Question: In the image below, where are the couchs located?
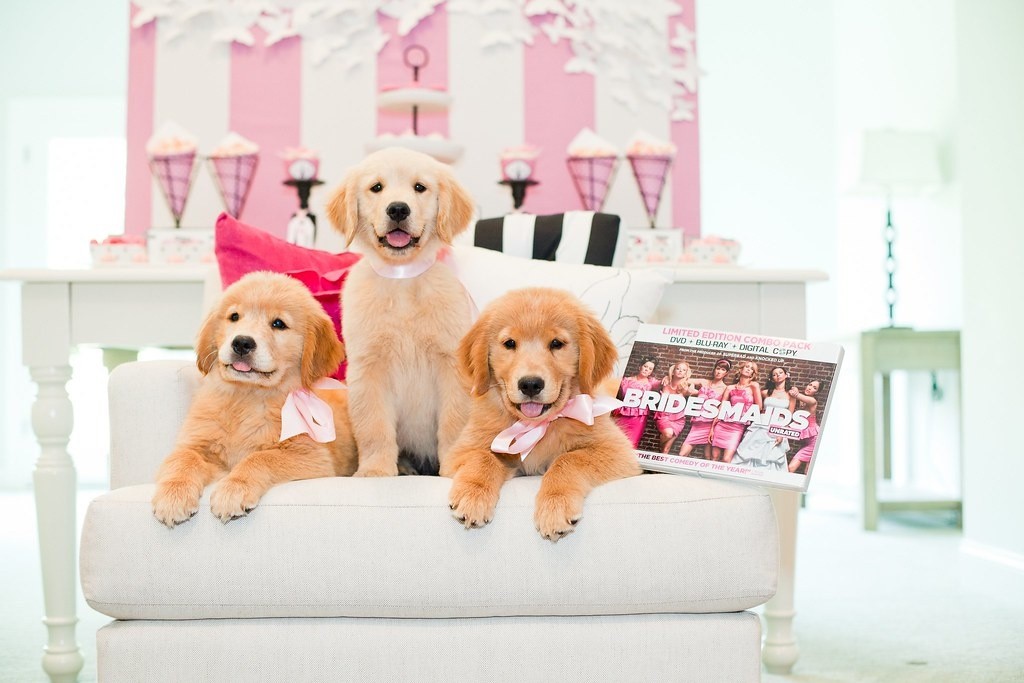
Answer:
[79,358,770,681]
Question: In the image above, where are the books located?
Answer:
[609,324,844,494]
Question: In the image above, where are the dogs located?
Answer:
[446,288,643,544]
[151,271,357,531]
[324,147,474,479]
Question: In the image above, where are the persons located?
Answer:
[654,361,699,454]
[731,367,796,473]
[789,379,825,474]
[708,361,763,463]
[661,359,732,460]
[612,356,663,450]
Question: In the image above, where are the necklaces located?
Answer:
[709,380,723,388]
[736,382,750,391]
[670,385,676,390]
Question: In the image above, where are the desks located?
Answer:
[0,256,826,683]
[862,328,963,533]
